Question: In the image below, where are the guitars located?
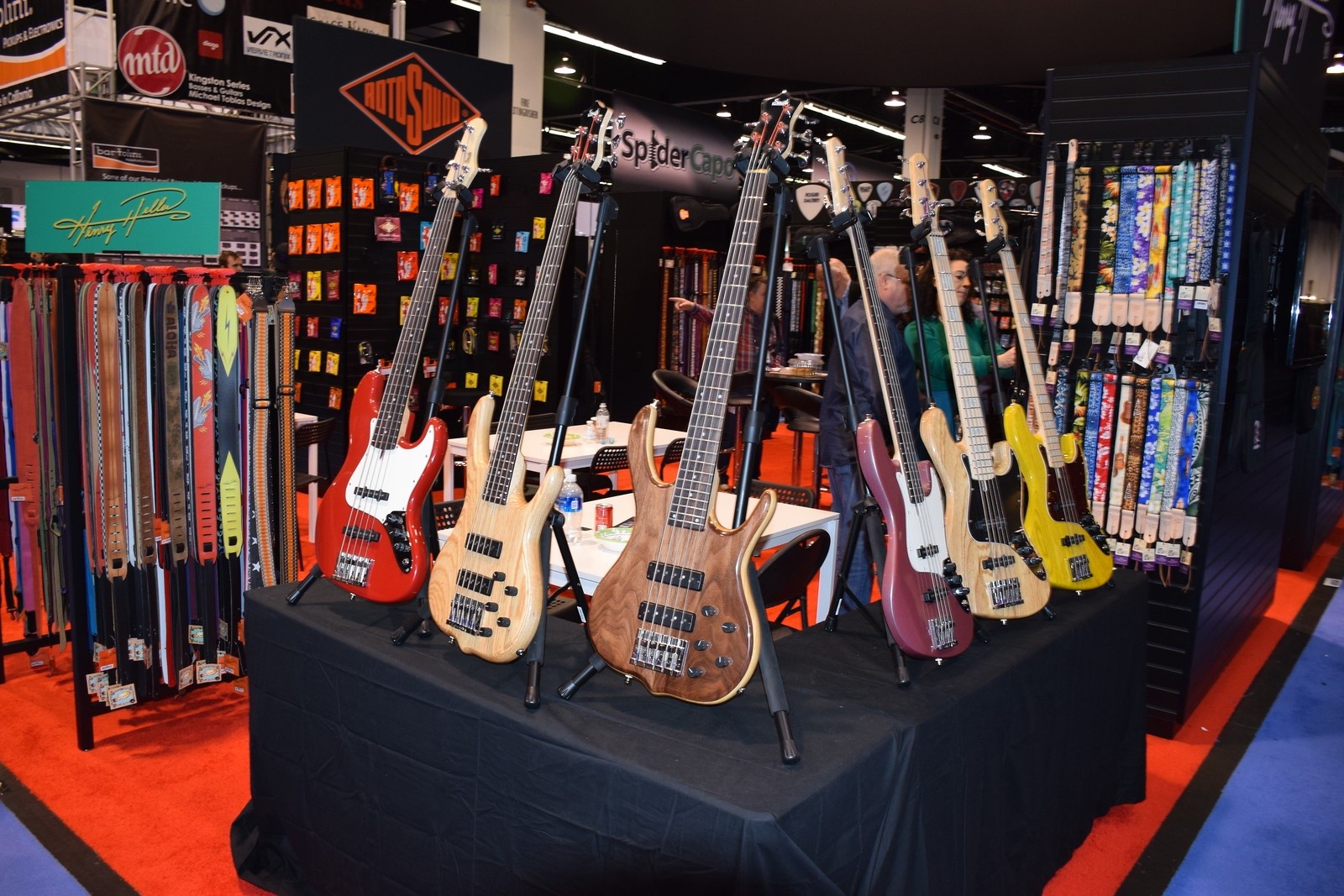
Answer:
[426,95,627,664]
[894,150,1050,619]
[585,88,816,706]
[968,176,1117,592]
[823,136,975,661]
[313,117,491,606]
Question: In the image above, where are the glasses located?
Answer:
[879,272,911,288]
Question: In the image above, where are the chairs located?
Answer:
[296,420,832,637]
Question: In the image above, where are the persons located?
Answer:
[818,248,923,615]
[904,249,1016,441]
[669,276,787,491]
[816,259,868,393]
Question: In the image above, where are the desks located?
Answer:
[437,488,841,625]
[444,420,688,501]
[231,566,1150,896]
[294,412,319,542]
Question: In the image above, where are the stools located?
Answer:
[777,384,826,509]
[651,369,770,488]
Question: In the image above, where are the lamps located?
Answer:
[982,163,1027,178]
[802,100,906,140]
[972,124,991,140]
[716,103,731,118]
[450,0,666,74]
[883,89,905,108]
